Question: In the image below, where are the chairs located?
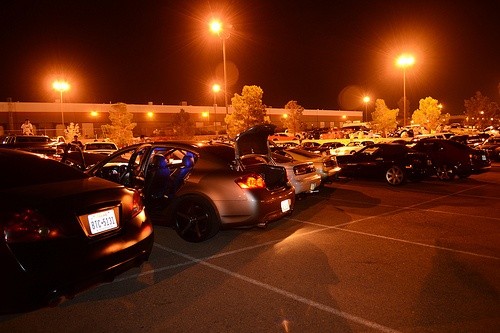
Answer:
[170,154,194,184]
[148,154,170,193]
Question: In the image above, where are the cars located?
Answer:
[230,122,500,228]
[84,138,297,243]
[0,133,154,307]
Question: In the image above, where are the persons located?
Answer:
[21,118,34,136]
[134,150,156,169]
[158,152,169,164]
[308,123,415,140]
[71,134,84,151]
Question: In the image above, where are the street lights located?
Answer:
[54,80,67,124]
[212,83,221,137]
[363,96,370,122]
[208,17,228,115]
[397,55,414,125]
